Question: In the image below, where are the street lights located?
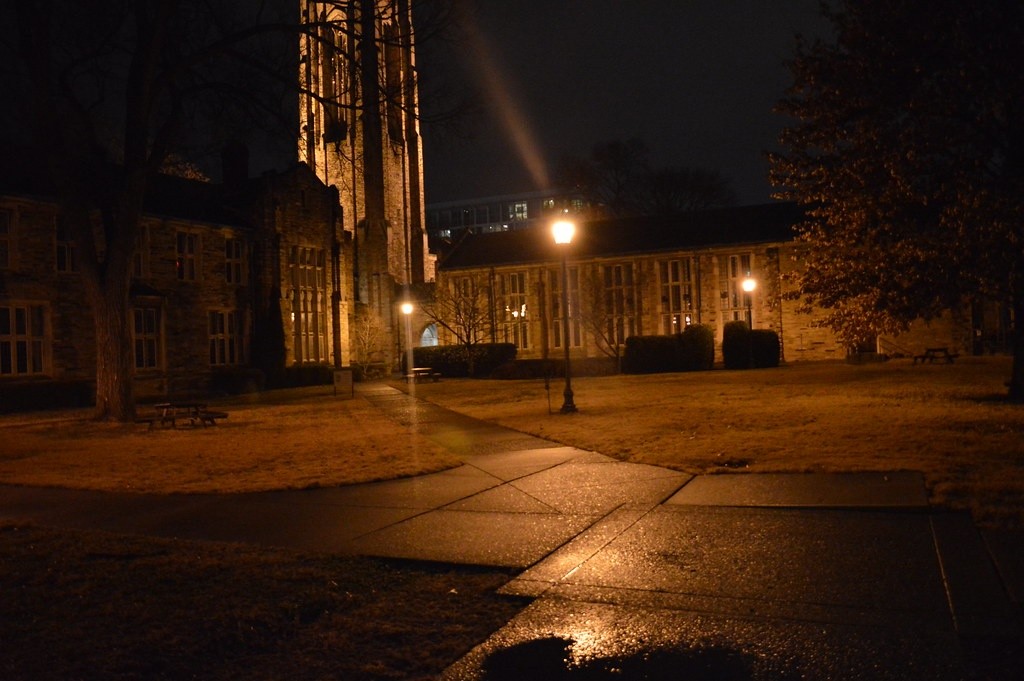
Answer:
[399,299,414,372]
[741,276,756,331]
[552,215,578,414]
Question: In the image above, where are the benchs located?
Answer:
[134,414,181,429]
[941,354,960,365]
[913,355,933,365]
[182,411,228,425]
[419,374,441,381]
[402,374,421,383]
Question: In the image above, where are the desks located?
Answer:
[154,402,207,428]
[921,348,953,365]
[410,368,433,382]
